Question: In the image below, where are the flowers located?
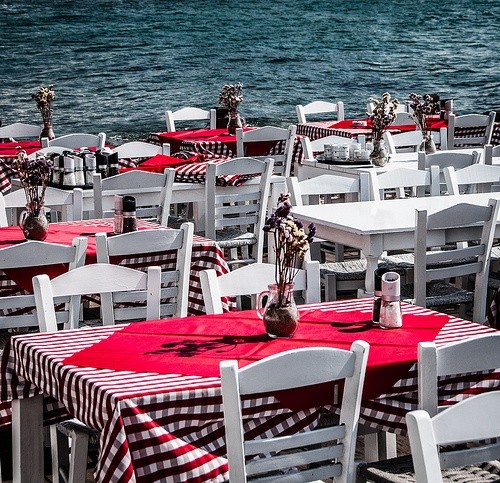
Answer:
[369,93,398,144]
[263,193,318,304]
[220,83,242,112]
[14,151,53,210]
[31,85,54,123]
[407,93,438,138]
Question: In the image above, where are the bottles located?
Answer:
[372,268,392,323]
[113,194,126,234]
[122,196,138,233]
[36,146,118,185]
[441,99,453,120]
[379,271,403,329]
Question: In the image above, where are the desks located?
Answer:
[147,126,309,178]
[291,193,500,295]
[0,141,118,195]
[296,119,448,141]
[1,300,500,483]
[0,220,231,343]
[11,171,286,234]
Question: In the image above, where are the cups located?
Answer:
[324,134,374,160]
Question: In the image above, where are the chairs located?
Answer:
[1,100,500,482]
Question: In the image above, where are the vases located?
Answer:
[367,137,389,169]
[227,112,247,136]
[417,134,437,155]
[256,282,300,339]
[40,124,56,146]
